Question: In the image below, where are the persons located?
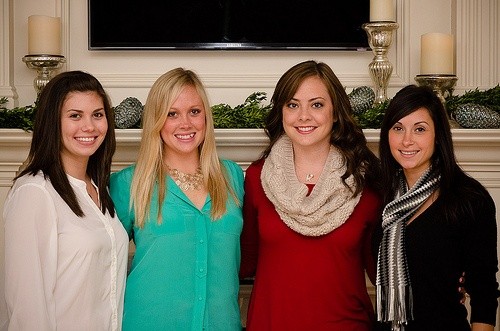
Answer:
[0,60,500,331]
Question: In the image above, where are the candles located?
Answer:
[370,0,396,23]
[420,33,454,76]
[27,14,62,56]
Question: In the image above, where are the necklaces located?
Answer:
[161,155,204,192]
[86,174,92,198]
[294,162,324,181]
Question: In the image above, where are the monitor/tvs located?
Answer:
[86,0,373,51]
